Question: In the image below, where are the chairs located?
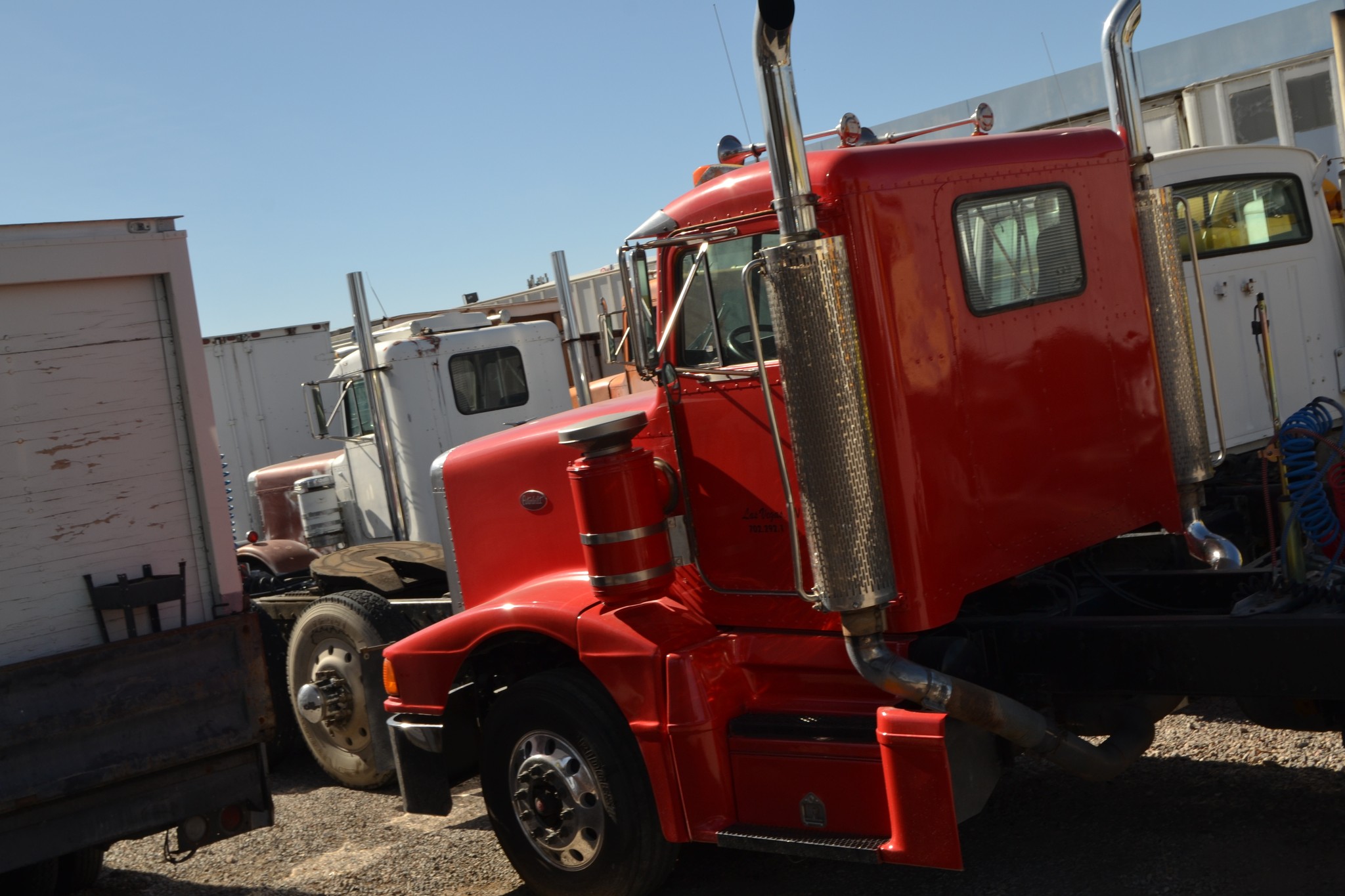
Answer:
[1174,216,1205,255]
[1033,224,1082,295]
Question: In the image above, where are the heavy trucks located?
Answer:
[236,0,1342,893]
